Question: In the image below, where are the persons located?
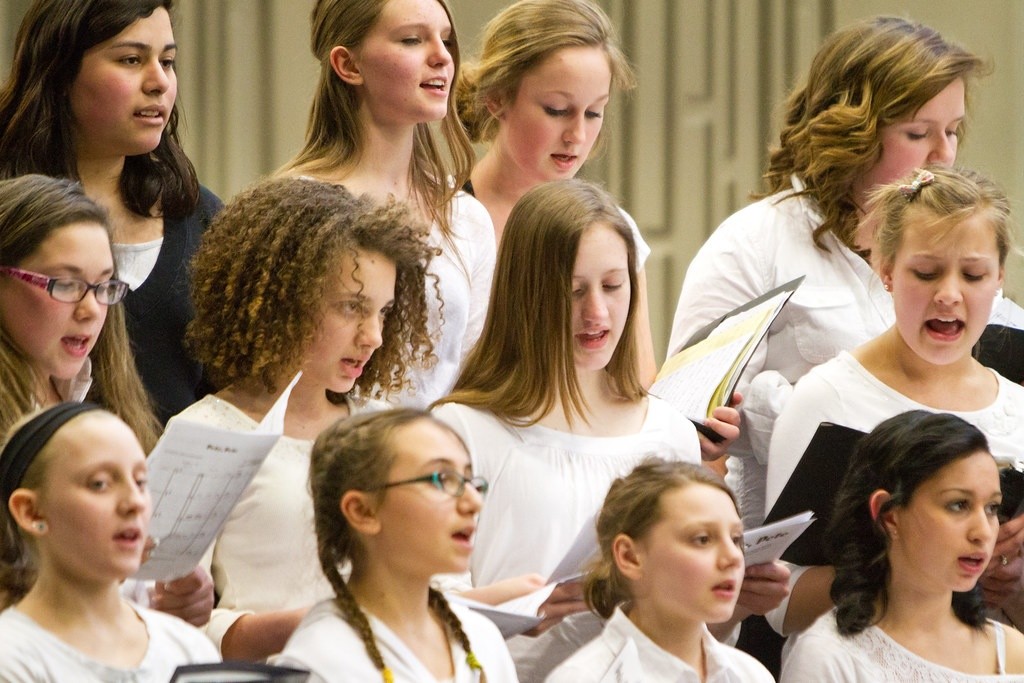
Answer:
[1,5,1024,679]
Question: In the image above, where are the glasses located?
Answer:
[364,471,489,502]
[0,265,130,305]
